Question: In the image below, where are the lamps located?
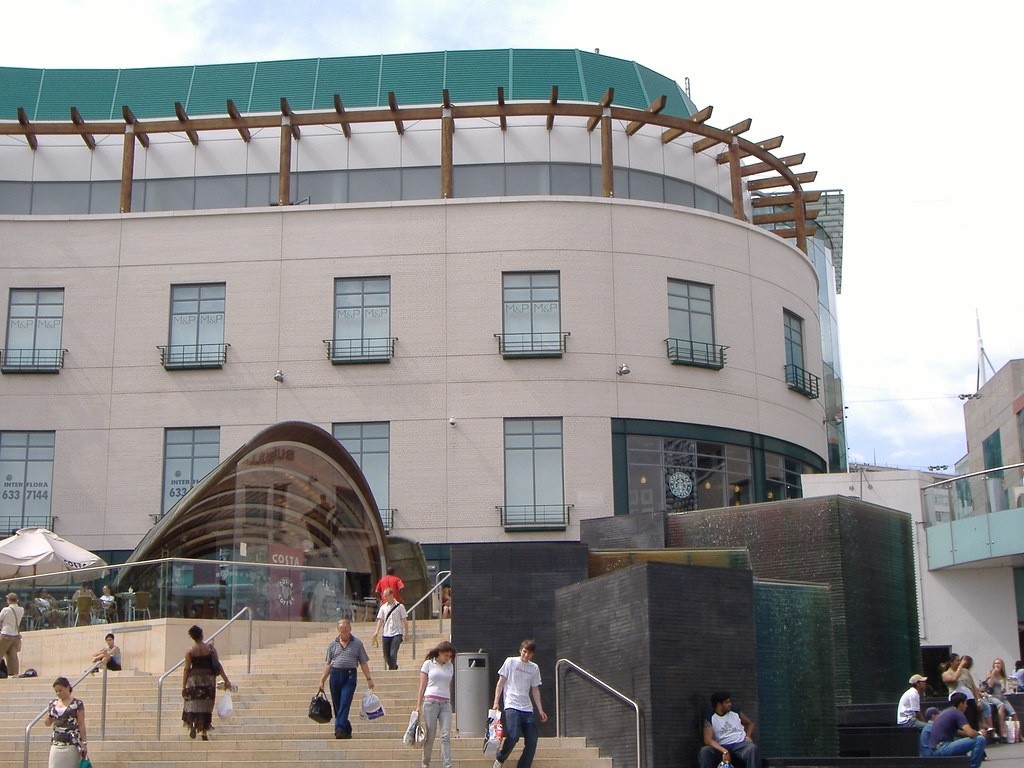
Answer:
[616,362,631,376]
[273,370,284,383]
[823,415,842,425]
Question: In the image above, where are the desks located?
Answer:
[55,599,78,627]
[115,592,151,621]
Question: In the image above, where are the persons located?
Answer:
[44,677,87,768]
[72,580,93,613]
[181,625,230,740]
[0,593,25,678]
[318,619,375,740]
[94,585,116,610]
[442,585,451,619]
[38,588,64,628]
[416,640,456,768]
[375,566,405,605]
[373,588,409,671]
[698,691,761,768]
[85,634,121,673]
[896,652,1024,768]
[492,639,547,768]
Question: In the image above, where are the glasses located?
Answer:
[956,659,961,661]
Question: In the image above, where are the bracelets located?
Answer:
[977,731,982,735]
[976,698,981,702]
[746,737,751,740]
[82,742,88,744]
[367,677,371,681]
[723,750,729,754]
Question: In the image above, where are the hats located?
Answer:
[909,674,927,684]
[925,707,940,718]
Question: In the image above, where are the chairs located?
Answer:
[20,591,152,631]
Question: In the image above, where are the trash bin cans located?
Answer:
[456,652,491,737]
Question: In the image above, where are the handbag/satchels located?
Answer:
[403,710,419,746]
[361,688,386,721]
[217,689,233,720]
[308,690,332,724]
[415,713,427,746]
[209,644,221,676]
[483,709,503,760]
[80,752,92,768]
[1005,720,1021,743]
[979,676,993,694]
[16,633,22,652]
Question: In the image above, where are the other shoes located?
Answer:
[992,732,1000,738]
[422,763,429,768]
[336,732,344,739]
[1021,736,1024,741]
[90,667,99,672]
[190,731,196,738]
[344,736,352,739]
[202,735,208,741]
[493,759,502,768]
[978,729,991,738]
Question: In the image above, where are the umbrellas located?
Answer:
[0,526,110,606]
